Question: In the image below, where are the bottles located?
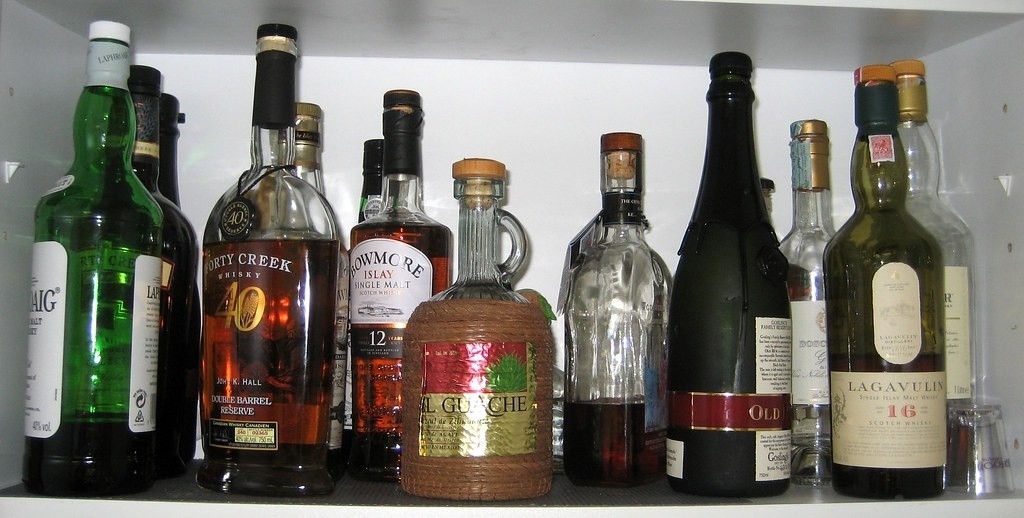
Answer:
[888,61,972,482]
[190,23,339,493]
[343,140,384,468]
[822,66,948,498]
[279,103,345,481]
[23,21,159,496]
[762,178,772,226]
[563,132,671,486]
[128,67,196,482]
[160,92,180,211]
[400,159,554,498]
[664,51,790,495]
[775,120,838,487]
[351,91,452,485]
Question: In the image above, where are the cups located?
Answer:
[946,406,1013,498]
